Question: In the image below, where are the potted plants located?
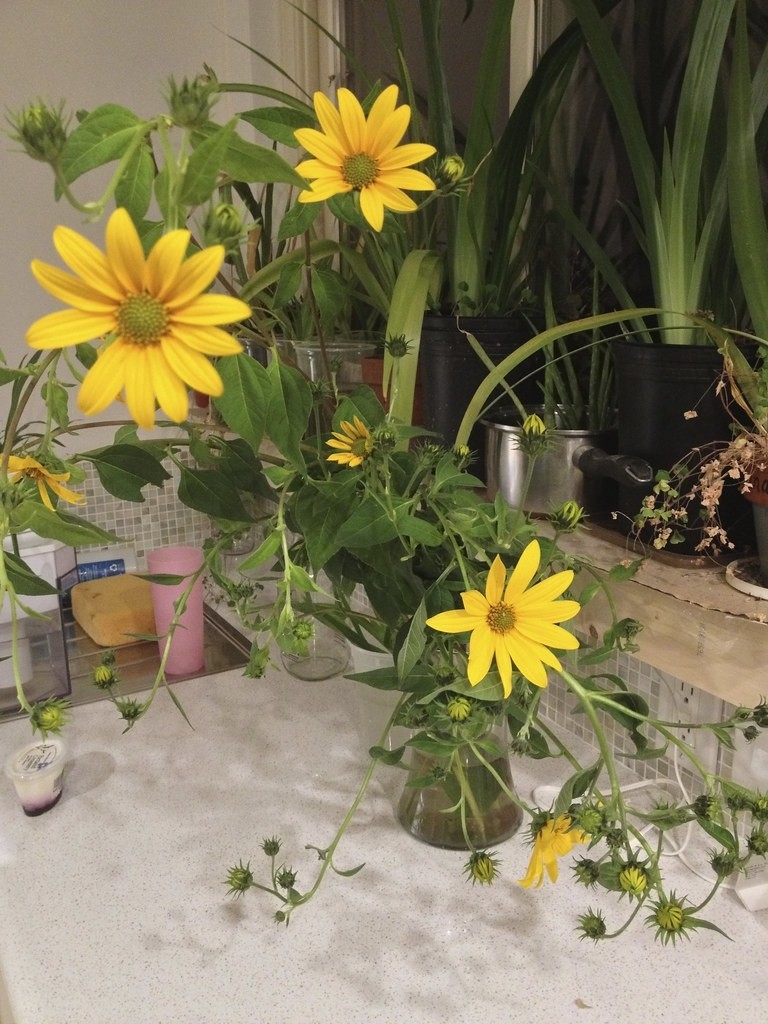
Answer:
[168,60,427,456]
[489,0,768,570]
[477,269,622,521]
[369,0,552,484]
[686,301,768,600]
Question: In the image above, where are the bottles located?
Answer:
[75,549,139,584]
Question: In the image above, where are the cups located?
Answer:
[147,547,203,676]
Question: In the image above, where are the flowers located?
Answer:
[0,67,768,946]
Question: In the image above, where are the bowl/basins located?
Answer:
[6,736,66,818]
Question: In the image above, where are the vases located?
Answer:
[393,690,523,850]
